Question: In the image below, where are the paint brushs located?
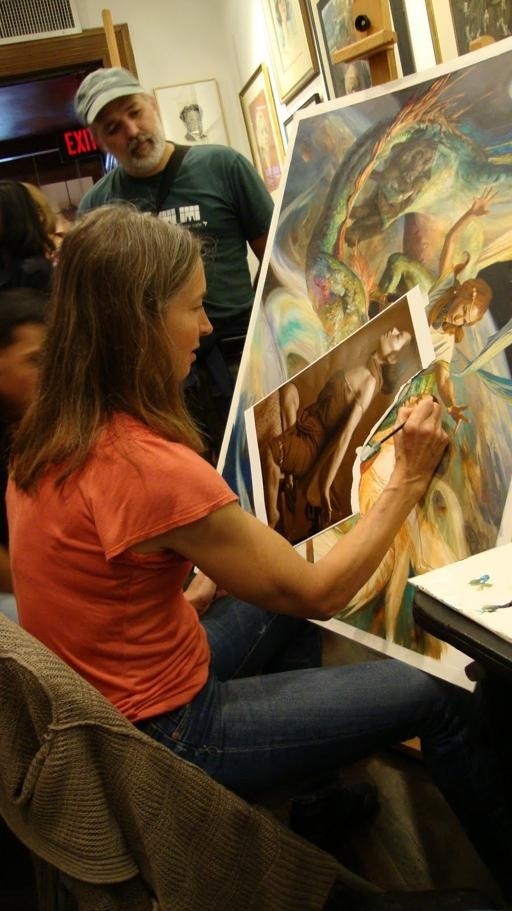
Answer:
[361,419,410,463]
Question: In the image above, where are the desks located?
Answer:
[413,540,512,673]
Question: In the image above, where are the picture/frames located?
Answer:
[146,0,321,201]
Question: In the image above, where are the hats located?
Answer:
[73,67,144,127]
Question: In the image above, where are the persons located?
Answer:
[266,328,416,527]
[1,182,56,292]
[73,69,275,452]
[179,104,207,139]
[378,184,500,429]
[0,293,53,619]
[251,111,278,182]
[4,205,504,879]
